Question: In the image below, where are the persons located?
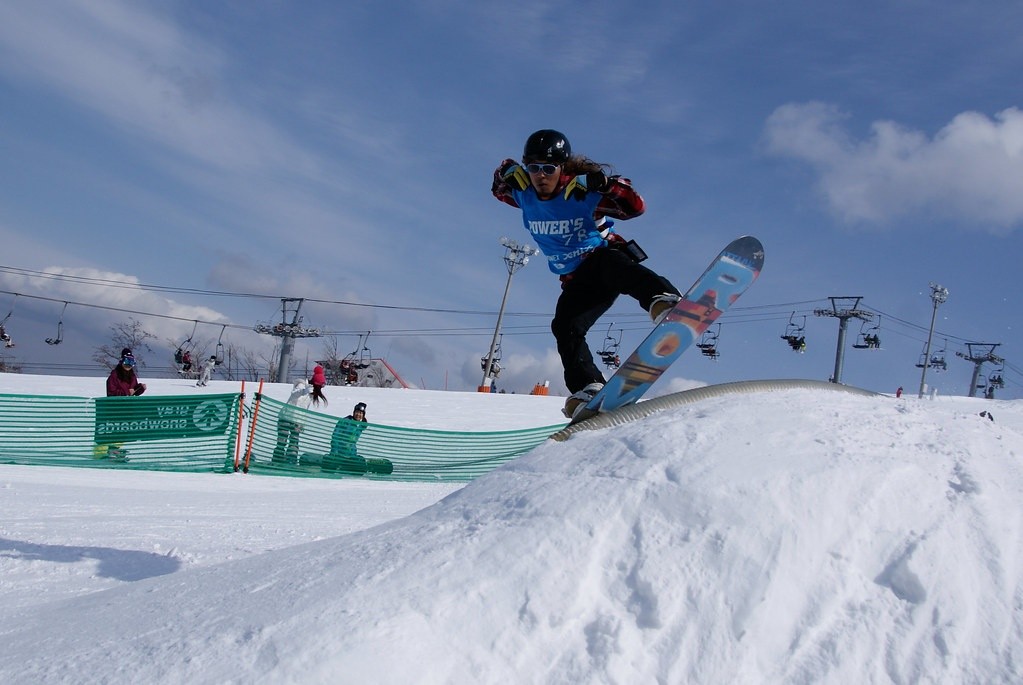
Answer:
[790,336,805,354]
[196,356,216,386]
[607,355,620,369]
[484,360,500,378]
[95,348,146,464]
[933,356,947,370]
[993,374,1004,389]
[489,129,682,418]
[174,349,192,373]
[896,386,903,398]
[340,360,358,384]
[0,325,14,348]
[980,411,993,421]
[708,347,717,360]
[322,403,367,476]
[271,366,328,470]
[865,333,879,348]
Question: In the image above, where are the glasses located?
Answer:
[122,358,135,366]
[354,405,365,411]
[526,164,558,176]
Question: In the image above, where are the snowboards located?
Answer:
[564,235,765,428]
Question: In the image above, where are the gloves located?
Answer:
[502,159,530,192]
[563,171,603,202]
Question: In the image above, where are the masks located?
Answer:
[211,359,214,361]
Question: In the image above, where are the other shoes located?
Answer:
[202,382,208,386]
[650,294,681,324]
[196,383,202,387]
[565,388,598,419]
[109,447,129,456]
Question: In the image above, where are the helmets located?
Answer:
[523,130,571,162]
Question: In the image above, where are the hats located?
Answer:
[120,347,133,364]
[312,365,327,387]
[355,403,367,417]
